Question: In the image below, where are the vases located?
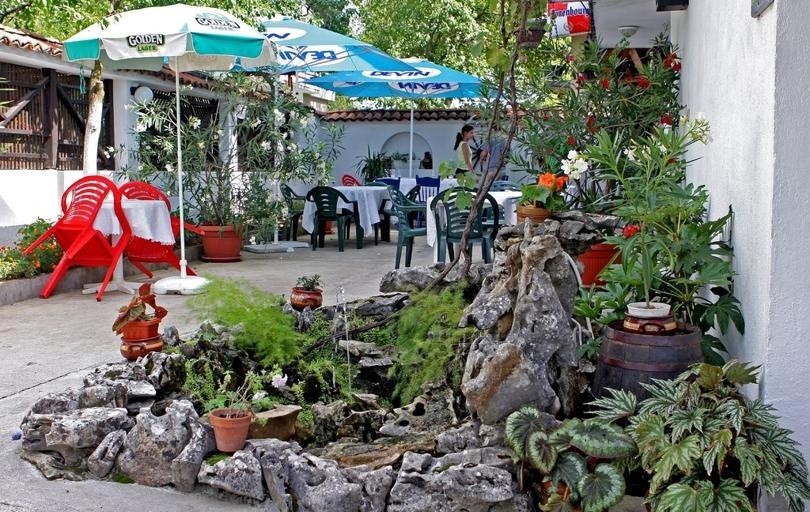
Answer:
[197,222,245,263]
[208,408,253,453]
[421,150,432,170]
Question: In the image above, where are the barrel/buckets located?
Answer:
[577,244,622,288]
[516,204,547,224]
[592,320,703,401]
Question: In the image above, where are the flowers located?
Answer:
[100,68,350,228]
[180,360,292,428]
[508,31,808,510]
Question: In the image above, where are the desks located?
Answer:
[423,186,523,266]
[67,196,177,299]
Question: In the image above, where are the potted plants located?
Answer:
[110,279,169,358]
[289,272,324,310]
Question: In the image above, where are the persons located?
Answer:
[454,126,473,173]
[480,124,512,181]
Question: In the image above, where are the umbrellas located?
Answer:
[167,15,421,245]
[62,2,274,279]
[302,56,505,179]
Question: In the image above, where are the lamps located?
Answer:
[230,101,250,121]
[128,84,155,106]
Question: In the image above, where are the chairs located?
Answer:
[432,186,501,264]
[23,173,132,301]
[273,169,458,270]
[480,179,521,260]
[115,173,207,279]
